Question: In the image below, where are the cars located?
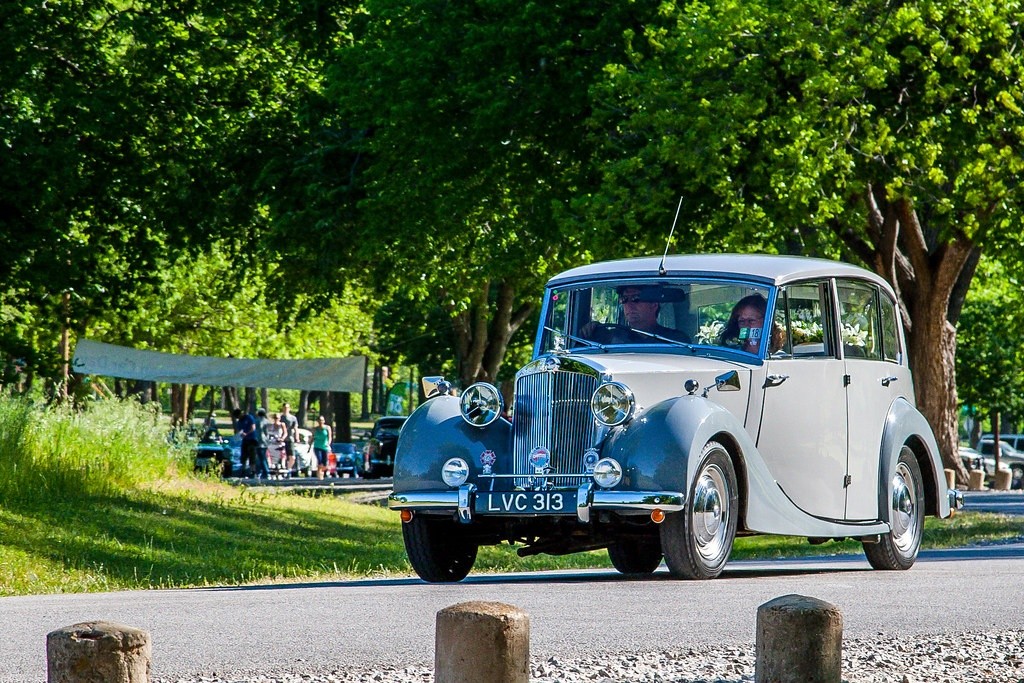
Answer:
[292,428,318,476]
[958,434,1024,482]
[360,415,408,480]
[331,442,360,478]
[387,253,965,580]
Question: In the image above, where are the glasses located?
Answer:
[619,294,640,303]
[736,318,762,324]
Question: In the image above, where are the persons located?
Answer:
[200,402,333,482]
[717,292,782,355]
[577,286,693,349]
